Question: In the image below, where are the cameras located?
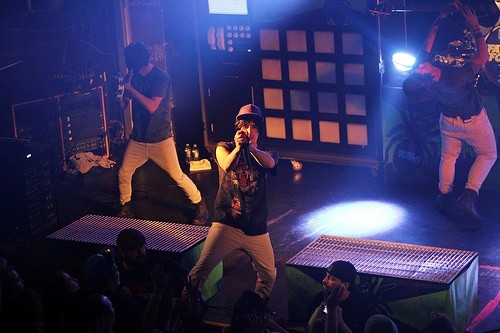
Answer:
[323,305,328,314]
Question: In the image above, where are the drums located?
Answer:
[478,26,500,91]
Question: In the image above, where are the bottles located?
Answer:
[185,144,199,163]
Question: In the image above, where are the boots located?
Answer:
[192,193,209,226]
[118,199,134,219]
[435,190,451,208]
[454,188,487,224]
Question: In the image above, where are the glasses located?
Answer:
[112,266,120,277]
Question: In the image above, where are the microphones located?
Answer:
[238,132,252,168]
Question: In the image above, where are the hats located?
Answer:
[319,261,357,282]
[236,104,265,123]
[83,251,113,289]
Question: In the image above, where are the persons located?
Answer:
[115,41,209,224]
[0,228,458,333]
[182,104,283,321]
[402,1,497,222]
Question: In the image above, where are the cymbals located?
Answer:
[113,71,124,102]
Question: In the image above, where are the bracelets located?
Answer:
[472,25,480,31]
[474,32,482,38]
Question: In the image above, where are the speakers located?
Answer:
[1,137,62,241]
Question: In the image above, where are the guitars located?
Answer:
[368,0,500,27]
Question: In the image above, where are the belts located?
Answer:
[441,110,473,119]
